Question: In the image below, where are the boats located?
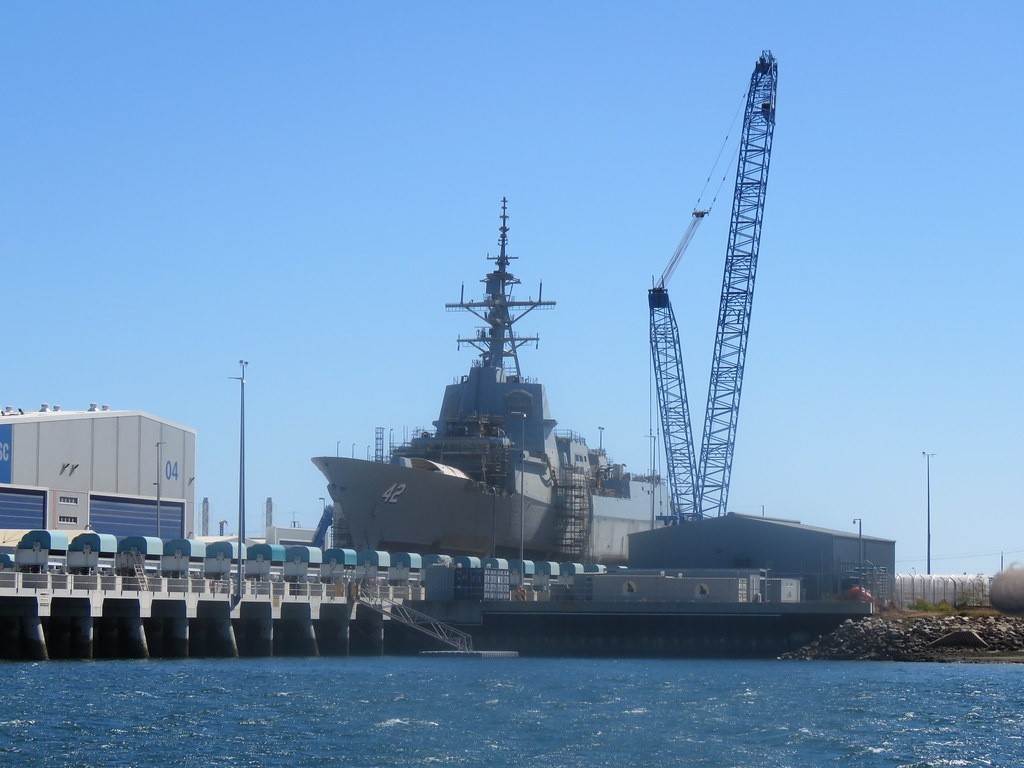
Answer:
[311,197,674,568]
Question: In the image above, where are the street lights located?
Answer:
[511,412,525,593]
[853,518,861,605]
[238,360,250,602]
[923,452,930,576]
[153,440,165,538]
